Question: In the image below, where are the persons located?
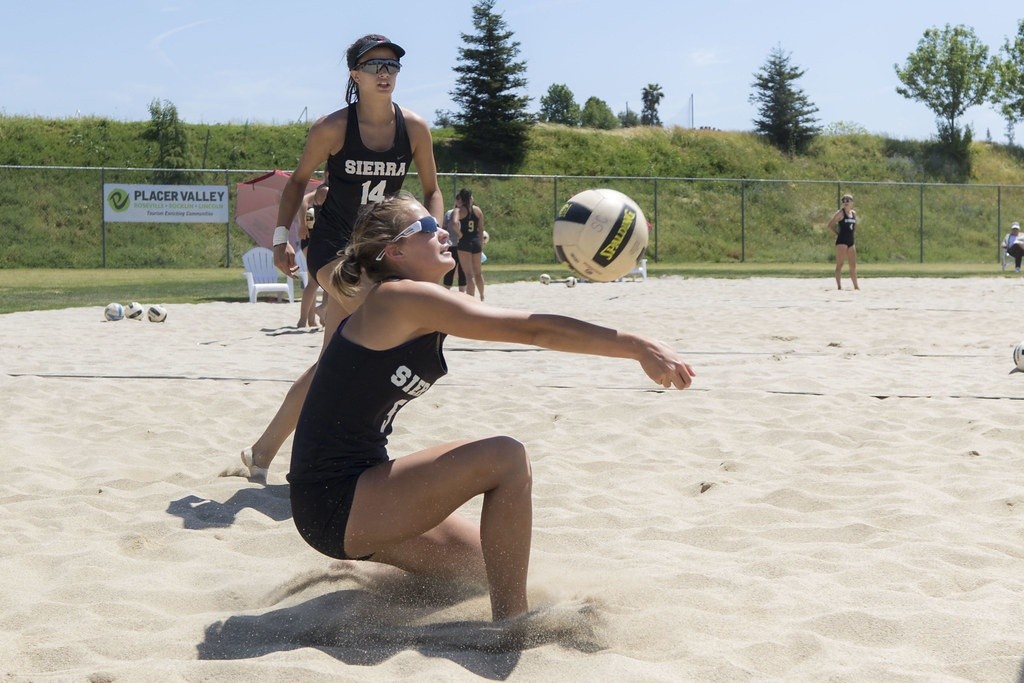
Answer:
[285,191,695,622]
[295,162,329,328]
[442,196,467,294]
[240,33,444,481]
[1002,222,1024,272]
[451,188,485,300]
[827,195,859,290]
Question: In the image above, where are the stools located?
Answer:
[618,258,647,281]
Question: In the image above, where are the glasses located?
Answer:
[374,217,440,265]
[351,57,403,76]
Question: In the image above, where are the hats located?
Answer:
[347,39,406,71]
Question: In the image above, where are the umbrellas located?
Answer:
[234,170,323,251]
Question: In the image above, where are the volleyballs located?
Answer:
[550,189,651,281]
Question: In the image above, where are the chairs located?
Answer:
[242,246,295,303]
[294,252,323,292]
[1002,247,1024,271]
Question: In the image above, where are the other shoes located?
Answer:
[240,447,270,481]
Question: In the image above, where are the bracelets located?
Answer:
[273,226,290,246]
